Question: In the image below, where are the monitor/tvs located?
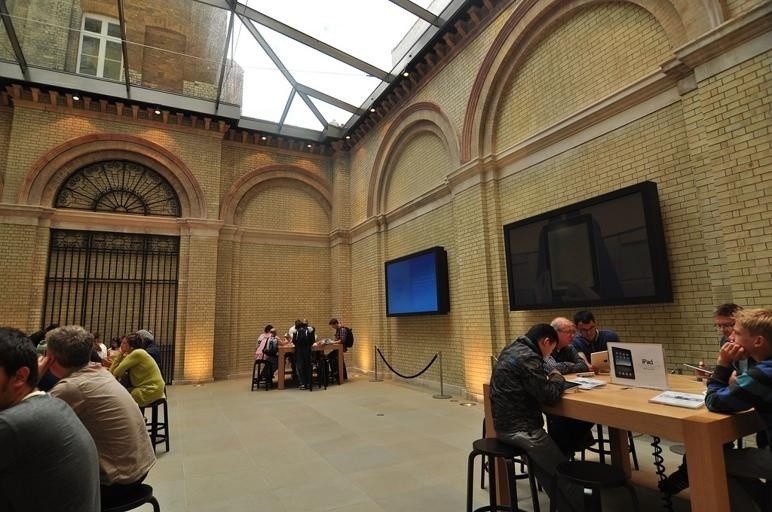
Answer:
[384,245,450,317]
[502,180,673,311]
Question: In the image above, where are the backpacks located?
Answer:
[262,337,277,355]
[344,326,353,347]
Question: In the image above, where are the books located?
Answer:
[648,390,706,409]
[562,370,608,394]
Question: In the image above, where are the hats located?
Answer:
[136,329,154,343]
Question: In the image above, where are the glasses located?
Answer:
[713,323,734,329]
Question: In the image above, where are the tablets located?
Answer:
[612,346,635,380]
[684,363,715,374]
[541,213,600,297]
[563,381,582,392]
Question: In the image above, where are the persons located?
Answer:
[658,303,758,495]
[327,319,347,382]
[489,323,586,512]
[255,319,318,390]
[572,311,621,375]
[544,316,595,452]
[705,307,771,512]
[0,322,167,512]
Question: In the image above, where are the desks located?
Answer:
[481,371,757,511]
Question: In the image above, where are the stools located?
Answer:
[466,438,542,511]
[100,479,162,512]
[481,413,541,491]
[251,340,344,395]
[582,419,640,470]
[138,397,172,453]
